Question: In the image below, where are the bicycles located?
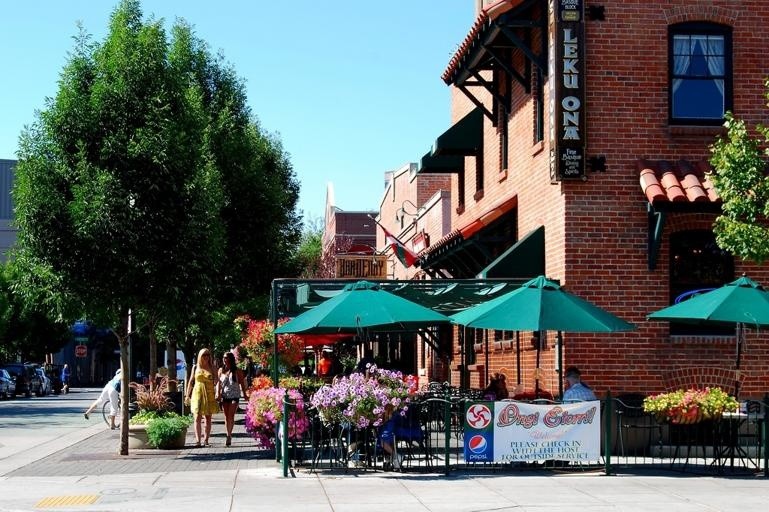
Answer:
[102,393,121,429]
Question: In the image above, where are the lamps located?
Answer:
[394,200,426,223]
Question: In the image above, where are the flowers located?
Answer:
[244,374,310,450]
[643,386,740,426]
[308,363,416,433]
[232,314,306,371]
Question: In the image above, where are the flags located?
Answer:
[380,226,419,269]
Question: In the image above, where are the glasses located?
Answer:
[201,352,212,357]
[222,356,228,360]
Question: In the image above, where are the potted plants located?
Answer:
[128,378,194,449]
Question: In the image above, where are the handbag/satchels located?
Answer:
[188,381,195,400]
[219,392,224,411]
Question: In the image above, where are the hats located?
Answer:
[562,366,579,376]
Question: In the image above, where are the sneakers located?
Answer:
[391,454,404,471]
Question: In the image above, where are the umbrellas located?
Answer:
[643,269,769,404]
[447,275,638,399]
[272,279,449,370]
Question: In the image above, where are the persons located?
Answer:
[561,366,598,403]
[376,407,424,471]
[331,374,343,388]
[83,366,122,430]
[184,347,219,447]
[214,351,251,446]
[60,363,72,395]
[479,372,510,400]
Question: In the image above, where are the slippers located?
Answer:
[110,426,115,430]
[83,412,89,420]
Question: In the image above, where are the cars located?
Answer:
[0,361,70,399]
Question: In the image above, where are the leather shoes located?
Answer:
[225,436,232,447]
[197,442,210,448]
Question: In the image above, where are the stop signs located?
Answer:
[75,345,87,357]
